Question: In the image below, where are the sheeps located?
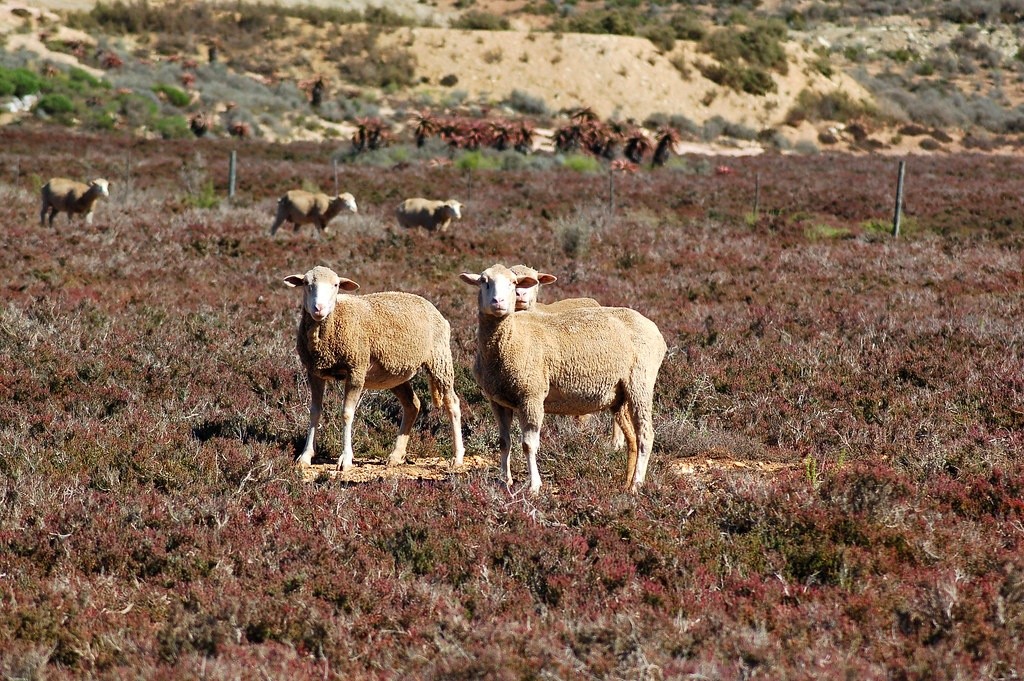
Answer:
[39,177,110,226]
[458,262,670,497]
[284,265,466,471]
[270,189,358,236]
[395,198,465,236]
